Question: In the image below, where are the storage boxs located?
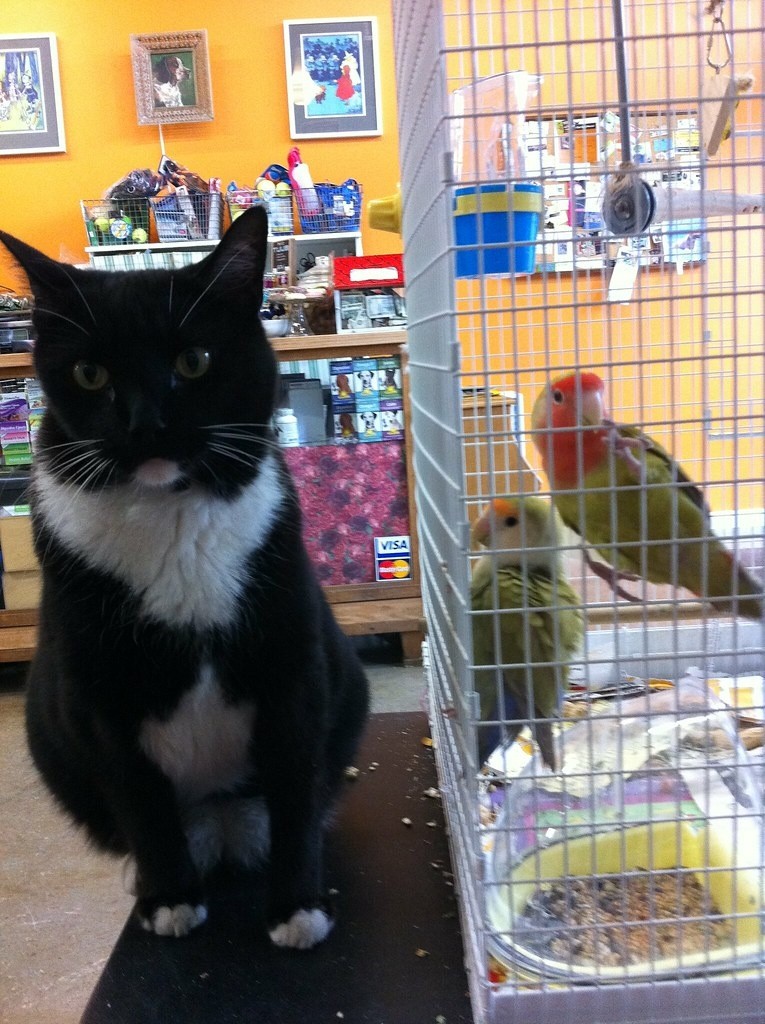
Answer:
[386,0,765,1024]
[327,361,355,405]
[378,357,403,401]
[79,197,150,245]
[380,399,404,441]
[223,189,295,236]
[293,183,365,234]
[332,404,358,443]
[279,443,409,586]
[352,359,379,402]
[330,253,405,289]
[0,504,43,610]
[355,401,383,442]
[0,477,31,505]
[149,193,226,241]
[0,380,47,465]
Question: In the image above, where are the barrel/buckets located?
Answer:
[453,184,543,278]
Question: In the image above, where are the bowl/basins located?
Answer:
[261,319,289,338]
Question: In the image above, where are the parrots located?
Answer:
[457,373,763,767]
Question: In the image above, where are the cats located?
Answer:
[1,203,368,958]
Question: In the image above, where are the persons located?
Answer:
[307,50,363,108]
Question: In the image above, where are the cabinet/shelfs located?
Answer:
[271,331,422,601]
[86,228,363,334]
[0,352,41,628]
[464,380,544,521]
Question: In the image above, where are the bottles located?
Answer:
[274,407,299,447]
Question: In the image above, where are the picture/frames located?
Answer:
[0,31,68,156]
[281,16,388,141]
[129,26,215,127]
